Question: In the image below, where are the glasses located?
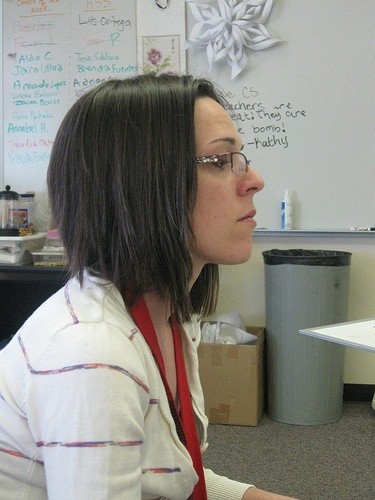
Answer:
[196,152,251,177]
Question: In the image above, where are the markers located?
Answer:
[351,225,374,232]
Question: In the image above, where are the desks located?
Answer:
[299,318,375,354]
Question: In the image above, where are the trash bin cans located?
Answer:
[262,249,352,425]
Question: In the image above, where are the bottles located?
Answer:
[280,188,294,231]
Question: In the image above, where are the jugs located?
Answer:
[0,185,20,237]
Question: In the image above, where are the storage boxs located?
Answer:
[0,232,48,267]
[31,251,68,266]
[197,326,266,426]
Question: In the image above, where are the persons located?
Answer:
[0,74,298,500]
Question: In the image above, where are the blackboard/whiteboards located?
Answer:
[0,0,375,239]
